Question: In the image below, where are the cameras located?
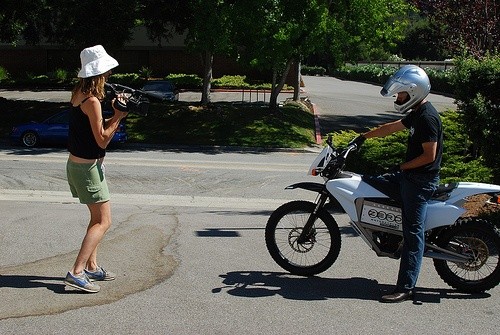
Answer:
[102,83,164,117]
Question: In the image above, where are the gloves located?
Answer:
[383,165,401,179]
[347,134,366,152]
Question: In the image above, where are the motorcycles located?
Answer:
[264,135,500,291]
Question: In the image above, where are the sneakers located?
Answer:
[84,266,116,281]
[64,271,100,292]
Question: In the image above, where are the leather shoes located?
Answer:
[380,287,412,302]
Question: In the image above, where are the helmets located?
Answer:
[380,65,431,114]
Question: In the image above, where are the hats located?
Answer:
[77,45,119,78]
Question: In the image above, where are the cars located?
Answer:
[138,81,179,108]
[11,109,129,148]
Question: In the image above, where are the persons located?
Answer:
[348,64,443,303]
[63,44,135,293]
